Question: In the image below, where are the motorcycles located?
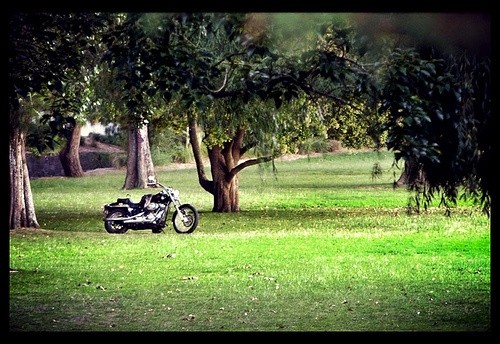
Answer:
[101,178,198,234]
